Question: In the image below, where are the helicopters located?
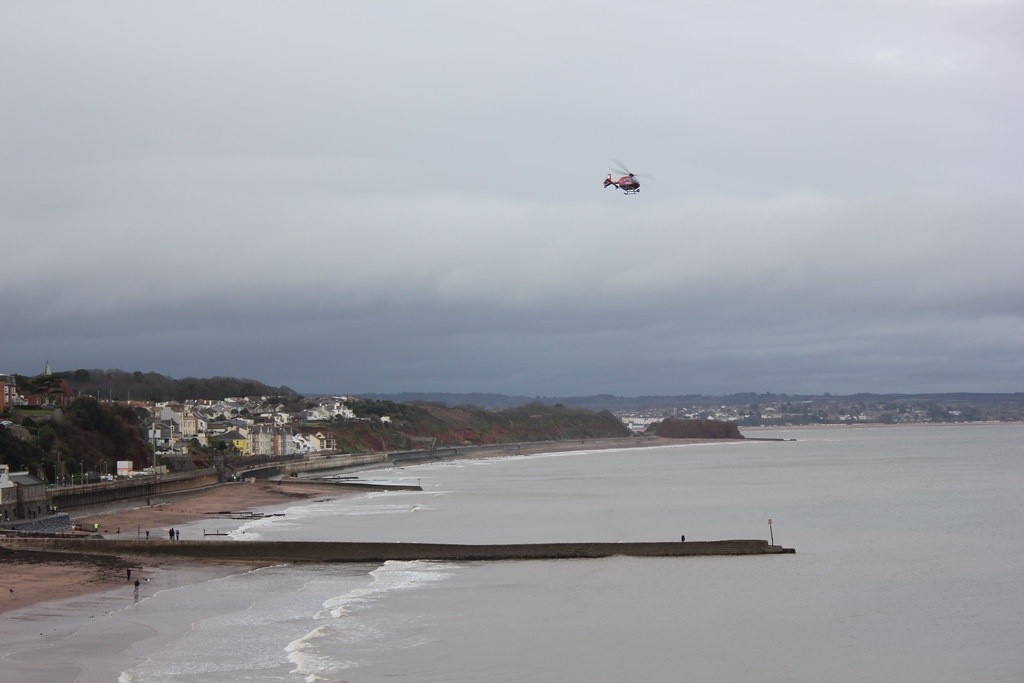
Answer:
[600,158,644,196]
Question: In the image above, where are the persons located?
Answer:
[51,505,179,592]
